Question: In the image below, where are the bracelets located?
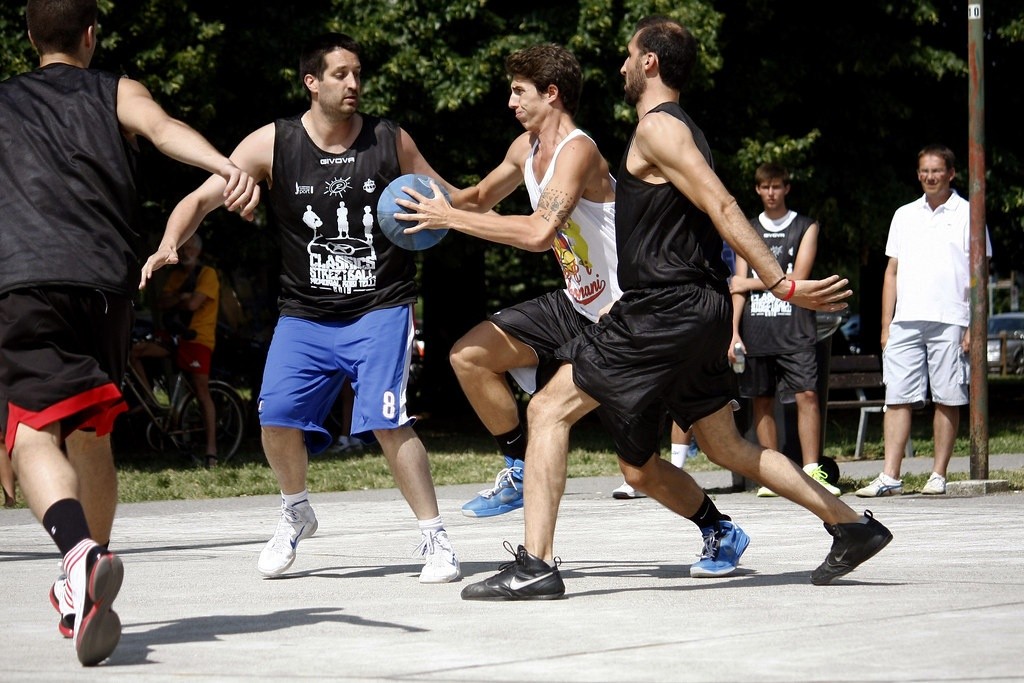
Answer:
[781,280,795,301]
[768,276,786,291]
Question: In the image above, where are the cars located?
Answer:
[986,312,1024,375]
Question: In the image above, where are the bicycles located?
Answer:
[120,333,248,470]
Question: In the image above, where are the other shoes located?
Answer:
[205,454,218,471]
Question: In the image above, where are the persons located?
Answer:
[395,43,750,579]
[124,232,220,469]
[728,161,841,498]
[326,377,363,453]
[855,145,992,497]
[138,32,502,583]
[0,0,260,669]
[462,16,893,600]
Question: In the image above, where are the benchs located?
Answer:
[828,352,930,457]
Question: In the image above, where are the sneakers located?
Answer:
[461,456,525,517]
[412,528,458,583]
[460,542,566,599]
[613,482,647,498]
[757,487,779,496]
[921,476,946,494]
[70,543,122,665]
[49,573,75,637]
[855,478,903,497]
[809,509,893,584]
[690,514,751,578]
[257,499,318,576]
[804,462,842,497]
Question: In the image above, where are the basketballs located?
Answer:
[377,174,452,249]
